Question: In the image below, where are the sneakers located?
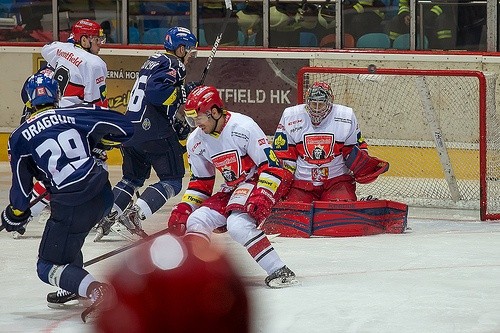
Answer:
[80,282,111,323]
[7,220,29,239]
[110,200,148,243]
[93,211,118,242]
[265,265,302,290]
[45,288,93,310]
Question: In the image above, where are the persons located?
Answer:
[168,85,295,289]
[20,17,109,221]
[262,81,408,241]
[0,72,134,323]
[93,26,199,239]
[94,236,250,333]
[0,0,485,50]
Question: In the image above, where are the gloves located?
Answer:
[1,204,35,235]
[341,146,390,184]
[245,188,276,221]
[168,202,192,238]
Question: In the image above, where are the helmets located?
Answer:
[163,27,198,51]
[303,81,334,126]
[318,4,336,29]
[93,236,251,333]
[20,73,59,109]
[72,19,103,43]
[185,85,223,127]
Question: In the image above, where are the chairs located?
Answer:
[100,9,429,50]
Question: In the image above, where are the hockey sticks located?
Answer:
[0,191,48,232]
[179,0,234,134]
[83,223,181,268]
